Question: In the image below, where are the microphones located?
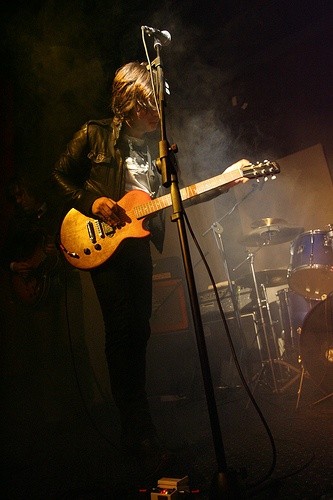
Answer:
[143,26,171,47]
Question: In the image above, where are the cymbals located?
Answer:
[246,217,288,232]
[239,225,306,247]
[234,269,287,288]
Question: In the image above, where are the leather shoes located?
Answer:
[115,410,172,459]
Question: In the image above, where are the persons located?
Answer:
[0,168,92,396]
[49,61,255,465]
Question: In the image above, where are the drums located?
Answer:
[289,228,333,300]
[299,295,333,402]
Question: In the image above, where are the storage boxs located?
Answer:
[144,278,267,399]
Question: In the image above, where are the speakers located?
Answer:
[145,329,205,404]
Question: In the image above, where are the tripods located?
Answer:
[232,241,310,412]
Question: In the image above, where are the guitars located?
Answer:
[60,159,281,271]
[9,247,62,307]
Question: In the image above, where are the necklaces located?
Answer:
[127,137,152,166]
